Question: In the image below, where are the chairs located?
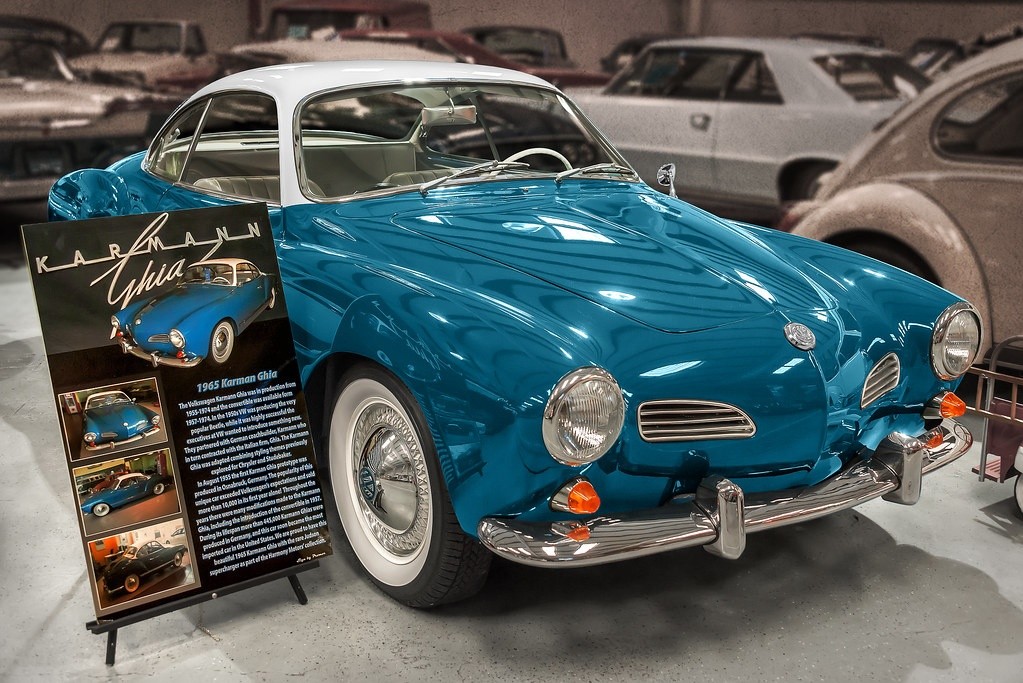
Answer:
[382,168,478,186]
[194,175,326,200]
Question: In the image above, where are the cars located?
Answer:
[77,474,106,493]
[462,24,612,88]
[777,39,1023,396]
[67,17,220,85]
[0,15,181,223]
[48,58,984,609]
[109,258,277,368]
[103,539,186,593]
[165,527,188,551]
[80,390,160,450]
[221,38,468,124]
[81,473,172,517]
[564,36,934,218]
[331,29,610,90]
[90,471,127,495]
